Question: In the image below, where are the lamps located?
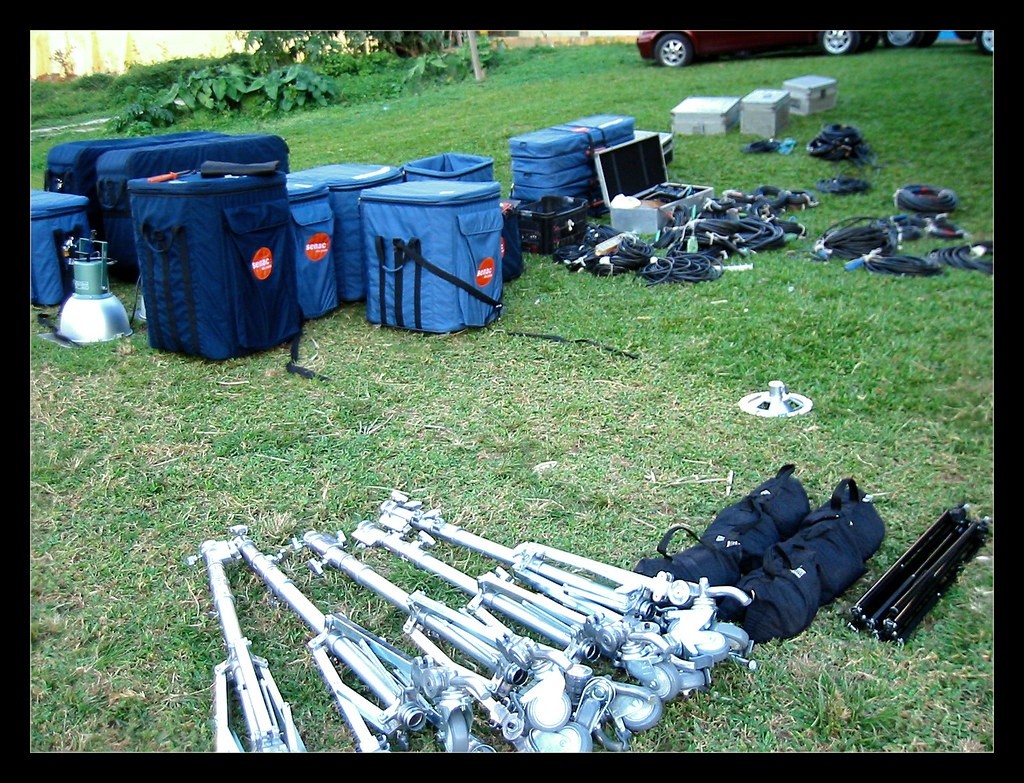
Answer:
[57,228,133,345]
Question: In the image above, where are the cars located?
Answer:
[636,30,879,68]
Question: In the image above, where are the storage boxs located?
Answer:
[669,96,742,138]
[595,133,715,233]
[520,194,588,255]
[782,75,837,116]
[738,87,790,139]
[636,130,674,164]
